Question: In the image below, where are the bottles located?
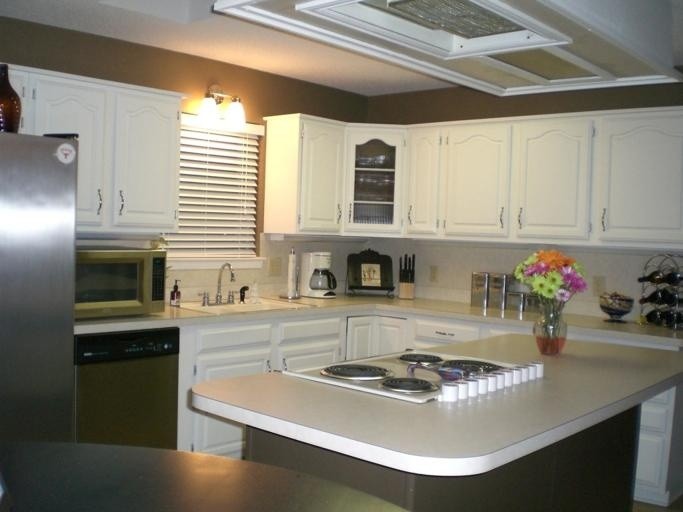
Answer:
[638,269,683,327]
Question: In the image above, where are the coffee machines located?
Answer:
[298,250,337,299]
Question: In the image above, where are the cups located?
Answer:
[491,373,505,389]
[483,374,497,392]
[510,368,521,384]
[442,383,458,401]
[533,363,544,378]
[468,381,478,396]
[454,381,468,399]
[478,377,488,394]
[518,366,529,382]
[500,370,513,387]
[526,365,537,380]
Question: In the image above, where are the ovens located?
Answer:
[73,327,180,450]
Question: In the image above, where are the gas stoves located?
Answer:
[290,354,504,403]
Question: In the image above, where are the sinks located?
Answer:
[181,297,306,314]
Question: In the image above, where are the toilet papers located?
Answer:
[285,247,299,299]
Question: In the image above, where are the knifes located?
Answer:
[399,254,415,282]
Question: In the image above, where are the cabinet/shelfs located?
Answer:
[344,304,412,362]
[442,111,596,246]
[630,335,683,508]
[1,59,189,235]
[404,120,442,241]
[412,309,480,350]
[596,105,683,250]
[261,112,403,239]
[177,306,344,461]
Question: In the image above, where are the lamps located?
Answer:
[199,82,246,131]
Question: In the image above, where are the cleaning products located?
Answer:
[170,278,184,306]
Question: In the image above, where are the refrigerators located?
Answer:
[1,132,79,446]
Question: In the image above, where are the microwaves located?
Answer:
[74,247,167,319]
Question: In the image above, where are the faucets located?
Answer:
[216,262,237,305]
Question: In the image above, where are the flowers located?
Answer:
[514,249,588,336]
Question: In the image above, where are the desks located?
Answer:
[187,331,683,512]
[1,439,414,512]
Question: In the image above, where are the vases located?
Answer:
[533,302,568,355]
